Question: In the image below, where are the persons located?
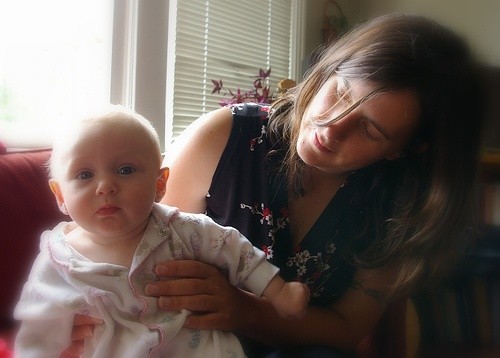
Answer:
[11,103,311,358]
[146,11,483,357]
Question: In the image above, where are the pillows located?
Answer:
[1,148,72,328]
